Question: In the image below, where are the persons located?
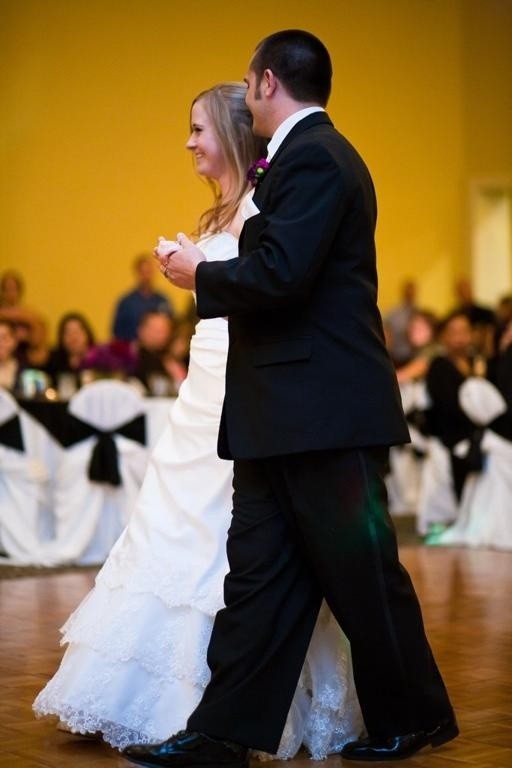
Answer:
[111,250,170,339]
[0,276,511,510]
[124,29,457,768]
[0,268,44,344]
[30,83,365,762]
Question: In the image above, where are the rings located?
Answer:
[158,264,168,274]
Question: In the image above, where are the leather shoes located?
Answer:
[342,719,459,761]
[122,728,248,766]
